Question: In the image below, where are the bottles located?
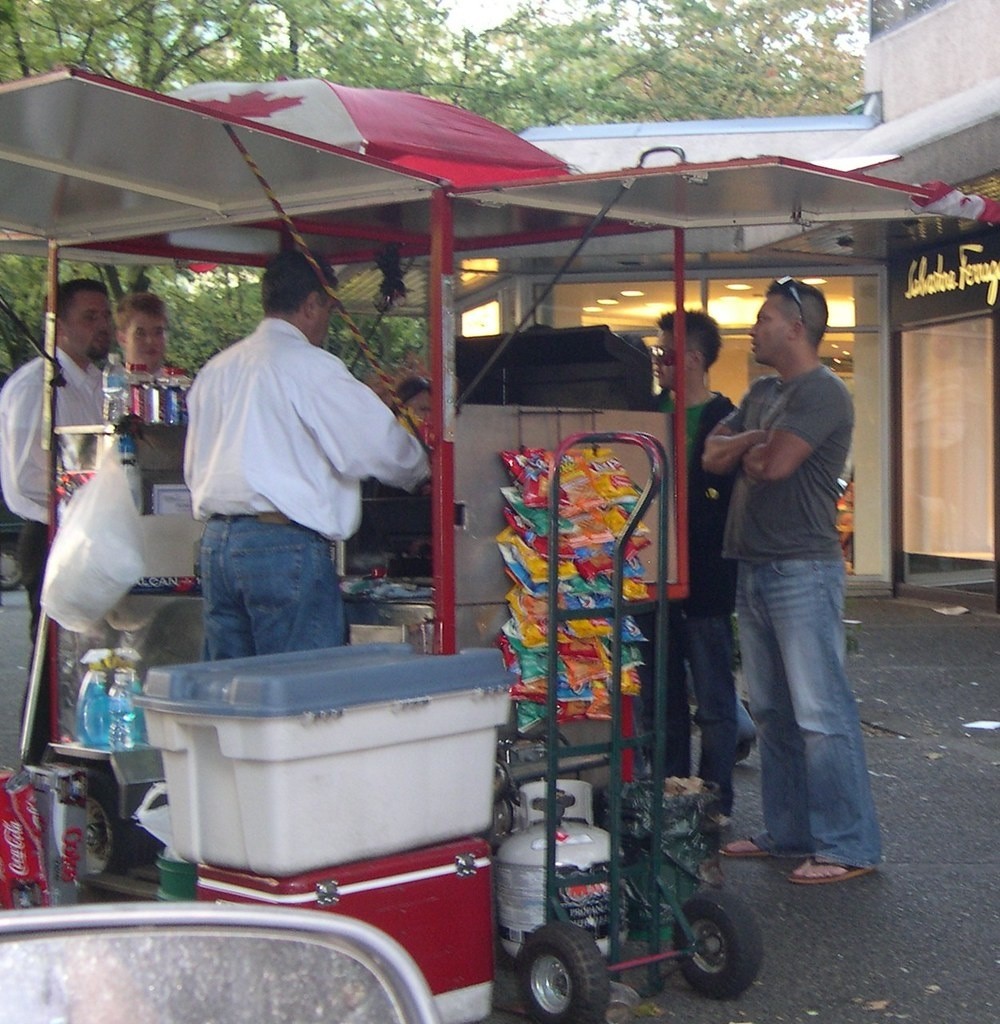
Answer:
[102,352,193,426]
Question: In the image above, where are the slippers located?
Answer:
[720,838,768,857]
[786,856,874,885]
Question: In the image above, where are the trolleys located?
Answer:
[487,429,766,1024]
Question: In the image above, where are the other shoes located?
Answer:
[719,816,730,833]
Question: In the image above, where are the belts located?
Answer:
[209,513,257,523]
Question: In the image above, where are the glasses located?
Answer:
[651,347,693,365]
[776,274,804,323]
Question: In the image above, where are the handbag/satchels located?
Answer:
[40,440,142,635]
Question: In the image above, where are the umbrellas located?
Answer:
[164,75,587,190]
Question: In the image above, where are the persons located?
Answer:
[0,278,170,764]
[182,248,432,663]
[701,275,882,885]
[633,310,740,827]
